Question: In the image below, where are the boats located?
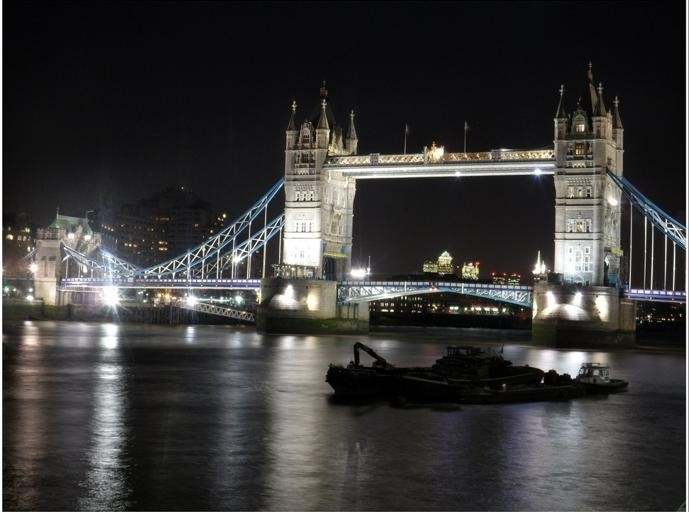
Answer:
[325,342,544,400]
[370,362,629,404]
